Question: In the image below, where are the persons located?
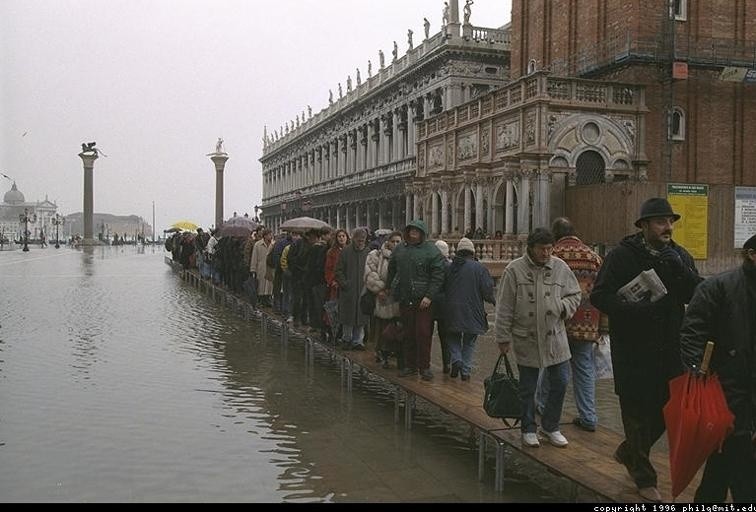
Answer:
[338,83,342,99]
[463,0,474,24]
[347,75,353,93]
[263,105,311,146]
[392,41,397,59]
[329,90,333,104]
[443,1,450,25]
[407,29,413,49]
[424,17,430,40]
[164,198,756,504]
[368,60,372,77]
[379,50,384,65]
[357,68,361,85]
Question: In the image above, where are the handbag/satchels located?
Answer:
[361,292,374,315]
[383,320,404,344]
[483,352,528,418]
[592,335,614,379]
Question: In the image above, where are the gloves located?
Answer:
[635,290,670,325]
[654,247,685,273]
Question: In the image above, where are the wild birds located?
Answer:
[0,172,13,181]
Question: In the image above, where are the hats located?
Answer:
[435,240,449,259]
[634,198,681,228]
[457,237,476,254]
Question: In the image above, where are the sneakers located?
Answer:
[258,296,366,350]
[444,360,471,380]
[573,415,596,431]
[376,349,434,380]
[521,404,569,447]
[613,450,662,503]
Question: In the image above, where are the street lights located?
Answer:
[18,208,37,251]
[52,213,65,248]
